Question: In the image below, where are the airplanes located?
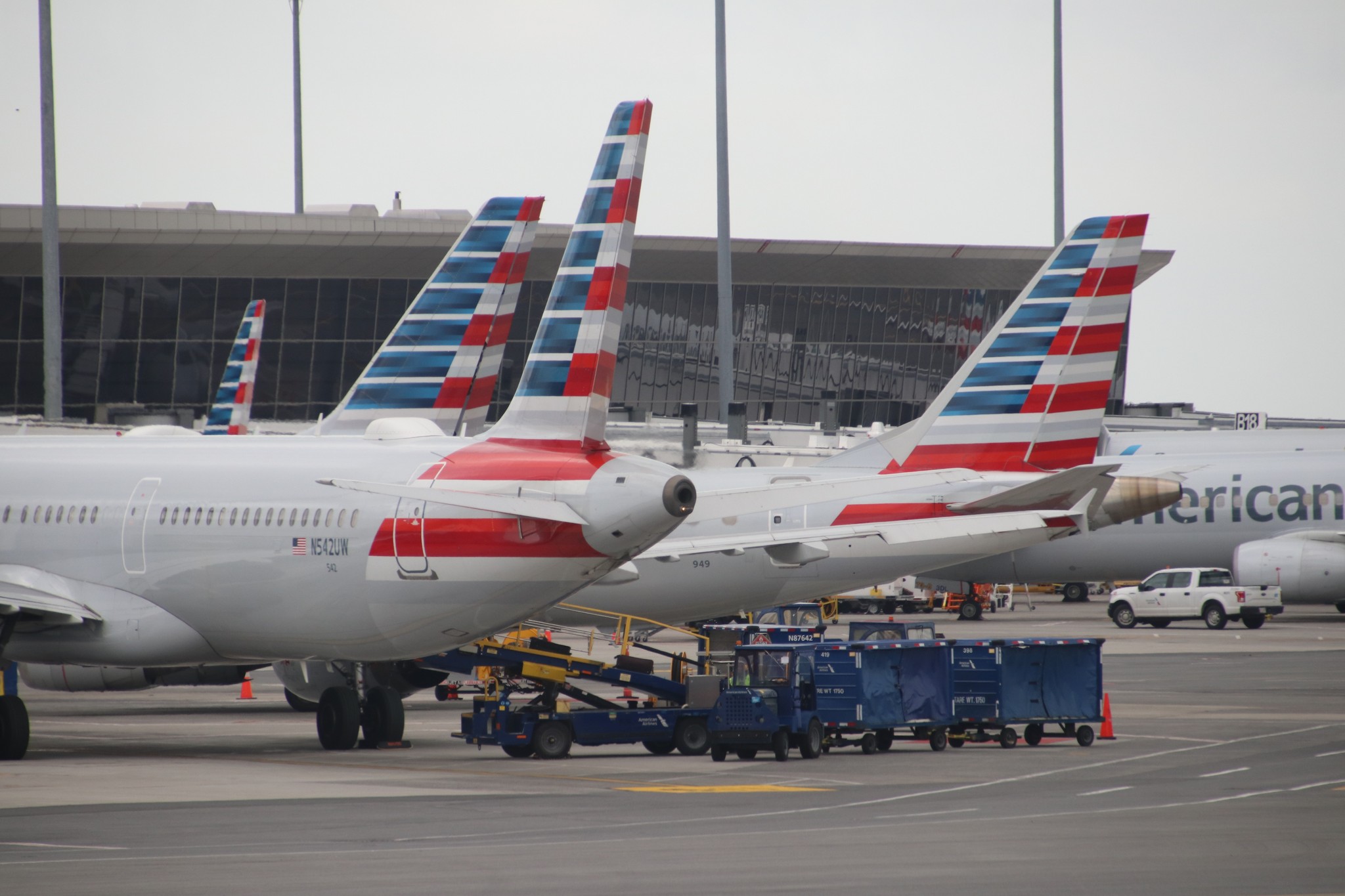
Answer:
[0,102,1345,763]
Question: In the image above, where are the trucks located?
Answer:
[705,643,826,762]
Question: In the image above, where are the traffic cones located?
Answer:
[608,633,622,645]
[544,627,552,642]
[447,684,463,700]
[1097,693,1117,740]
[235,672,257,699]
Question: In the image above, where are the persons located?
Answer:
[729,661,750,686]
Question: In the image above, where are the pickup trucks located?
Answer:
[1108,567,1284,629]
